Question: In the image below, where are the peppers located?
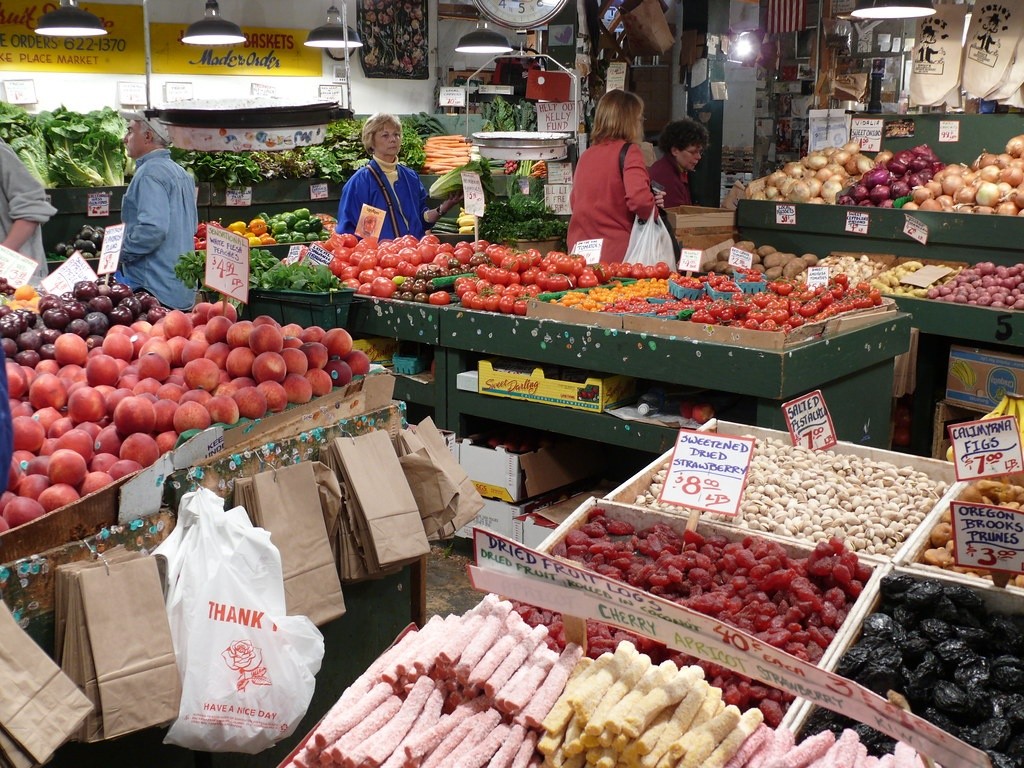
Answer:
[194,208,329,248]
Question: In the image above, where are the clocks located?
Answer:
[471,0,568,31]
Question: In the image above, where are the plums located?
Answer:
[0,279,171,367]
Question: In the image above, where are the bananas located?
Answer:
[946,394,1024,462]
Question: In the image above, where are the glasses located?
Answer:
[684,148,704,157]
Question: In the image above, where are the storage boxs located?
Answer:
[471,359,627,413]
[454,429,572,503]
[662,205,735,254]
[403,421,455,457]
[945,347,1024,414]
[887,326,919,450]
[352,243,896,353]
[453,492,610,549]
[274,416,1024,768]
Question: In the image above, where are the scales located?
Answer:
[452,0,589,178]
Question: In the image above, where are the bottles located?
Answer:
[635,386,668,416]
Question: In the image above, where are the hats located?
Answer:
[119,107,172,144]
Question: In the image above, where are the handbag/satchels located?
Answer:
[621,206,682,273]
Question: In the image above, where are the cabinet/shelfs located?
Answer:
[1,284,407,629]
[347,294,911,456]
[735,199,1024,350]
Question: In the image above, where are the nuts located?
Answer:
[636,432,951,557]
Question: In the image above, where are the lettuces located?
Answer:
[429,157,496,205]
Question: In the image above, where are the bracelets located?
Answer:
[435,204,447,216]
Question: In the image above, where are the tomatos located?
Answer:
[299,233,680,315]
[550,268,882,335]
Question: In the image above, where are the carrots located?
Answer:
[422,134,472,174]
[529,160,547,178]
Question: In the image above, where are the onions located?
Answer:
[751,134,1024,216]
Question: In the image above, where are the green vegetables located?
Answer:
[170,118,371,190]
[480,96,537,176]
[479,179,569,250]
[398,111,446,165]
[175,249,343,316]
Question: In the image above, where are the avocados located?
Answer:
[55,224,106,257]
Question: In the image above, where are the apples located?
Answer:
[681,398,714,423]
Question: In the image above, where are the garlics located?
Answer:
[797,254,886,286]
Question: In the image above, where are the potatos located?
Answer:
[930,262,1024,310]
[704,241,818,279]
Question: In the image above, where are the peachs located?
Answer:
[0,302,370,533]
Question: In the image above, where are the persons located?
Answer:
[118,106,198,313]
[335,113,465,240]
[647,115,709,208]
[565,88,666,264]
[0,135,58,277]
[0,339,16,502]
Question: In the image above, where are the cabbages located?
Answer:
[0,99,137,189]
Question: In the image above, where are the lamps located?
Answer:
[454,29,513,55]
[304,6,363,49]
[33,0,108,37]
[181,0,247,45]
[850,0,936,20]
